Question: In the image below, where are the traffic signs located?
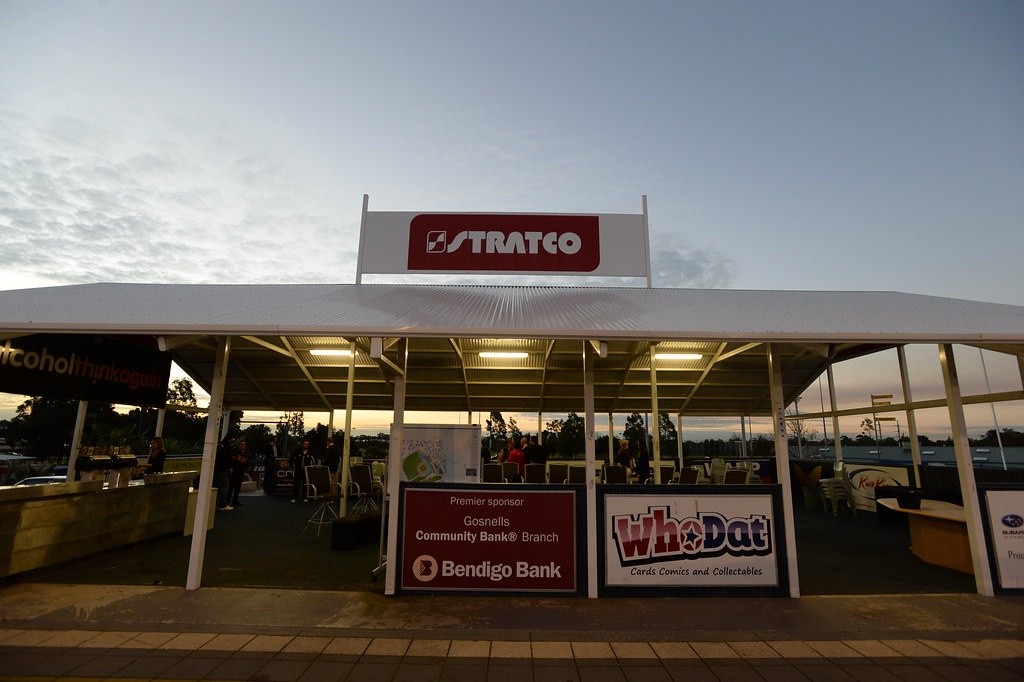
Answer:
[875,418,896,421]
[873,395,893,398]
[874,402,891,405]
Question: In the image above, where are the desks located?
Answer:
[876,498,975,575]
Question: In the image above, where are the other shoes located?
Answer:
[304,499,308,502]
[290,499,296,503]
[218,506,233,510]
[233,503,243,506]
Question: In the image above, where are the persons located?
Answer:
[147,437,166,474]
[497,435,547,477]
[321,437,340,505]
[288,439,316,503]
[635,439,649,484]
[214,430,248,511]
[263,436,279,495]
[617,439,635,469]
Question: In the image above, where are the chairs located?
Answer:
[819,461,848,516]
[302,450,750,537]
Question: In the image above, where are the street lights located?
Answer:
[794,396,803,459]
